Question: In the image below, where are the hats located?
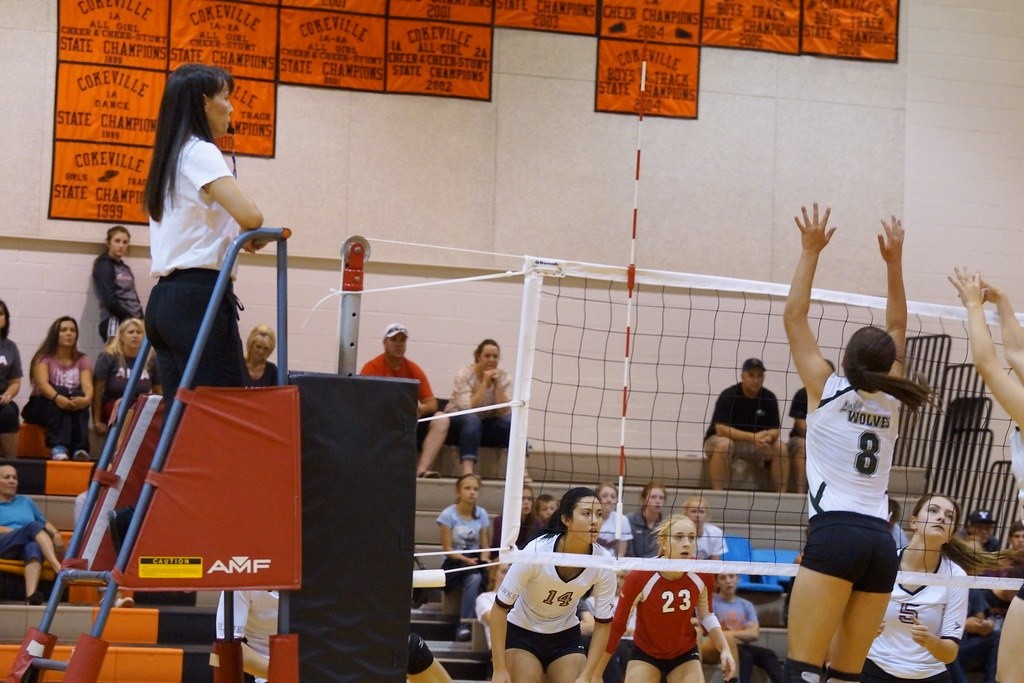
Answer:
[970,510,996,523]
[383,322,409,338]
[744,357,767,371]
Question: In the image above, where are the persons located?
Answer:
[445,338,534,483]
[434,471,494,641]
[108,64,265,556]
[945,264,1024,683]
[89,319,165,436]
[357,323,450,479]
[242,325,279,387]
[0,299,22,460]
[888,497,1024,683]
[780,204,935,683]
[478,481,762,683]
[1,462,65,607]
[860,491,1017,683]
[788,359,835,493]
[701,359,792,494]
[91,225,145,343]
[19,316,95,462]
[215,589,278,683]
[407,631,454,683]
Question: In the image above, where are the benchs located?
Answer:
[0,425,929,683]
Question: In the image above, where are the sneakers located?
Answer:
[74,450,90,461]
[457,626,472,641]
[26,591,44,605]
[420,470,440,479]
[51,446,69,461]
